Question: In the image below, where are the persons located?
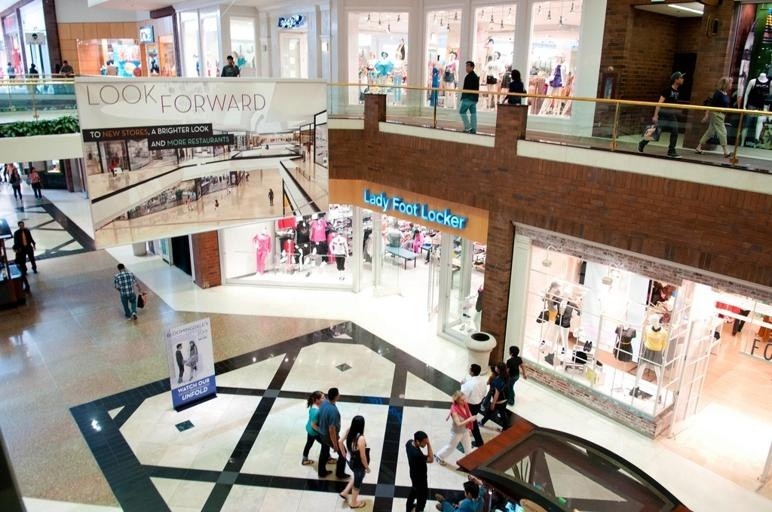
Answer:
[436,346,528,465]
[535,283,666,381]
[460,61,480,133]
[405,430,434,512]
[175,343,186,382]
[696,77,735,158]
[316,388,351,480]
[219,55,242,78]
[186,340,199,381]
[6,62,17,79]
[741,72,772,147]
[337,415,371,509]
[254,212,486,270]
[358,53,574,115]
[58,59,74,93]
[0,162,42,200]
[112,263,141,321]
[13,221,37,276]
[637,71,686,157]
[27,64,40,95]
[301,391,338,466]
[435,478,489,512]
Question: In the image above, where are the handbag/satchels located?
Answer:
[350,434,370,472]
[536,310,550,324]
[572,350,587,363]
[137,289,148,308]
[522,86,528,99]
[640,122,662,141]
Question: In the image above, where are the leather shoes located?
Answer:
[320,471,332,477]
[471,440,484,447]
[339,473,350,479]
[583,341,593,352]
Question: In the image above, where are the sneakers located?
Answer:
[435,493,445,511]
[128,312,138,322]
[463,122,477,133]
[24,267,39,276]
[177,376,193,384]
[630,386,639,396]
[435,454,448,466]
[496,425,504,432]
[638,143,644,152]
[476,419,485,427]
[667,150,684,159]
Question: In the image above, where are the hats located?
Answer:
[669,71,686,82]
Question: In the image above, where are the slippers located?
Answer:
[339,492,349,500]
[349,500,366,509]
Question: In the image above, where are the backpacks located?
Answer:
[702,95,713,106]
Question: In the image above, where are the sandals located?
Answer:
[302,459,314,466]
[695,149,705,155]
[724,153,735,159]
[328,458,338,464]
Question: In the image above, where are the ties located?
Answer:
[21,230,28,247]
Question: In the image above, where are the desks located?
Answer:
[385,245,432,270]
